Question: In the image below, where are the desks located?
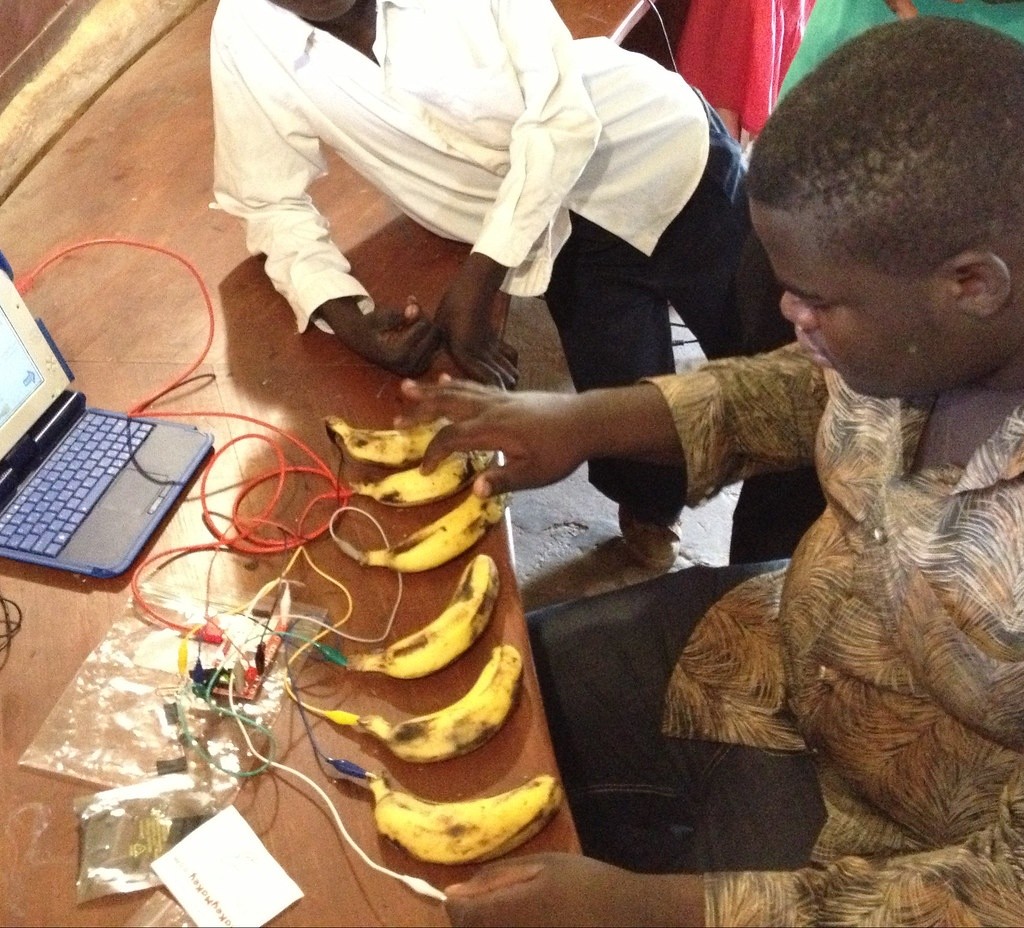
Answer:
[1,0,660,927]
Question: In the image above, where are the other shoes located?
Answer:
[619,503,683,570]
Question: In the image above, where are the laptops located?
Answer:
[0,250,215,580]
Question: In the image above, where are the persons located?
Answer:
[399,0,1024,928]
[204,0,825,571]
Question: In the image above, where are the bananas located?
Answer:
[326,417,564,865]
